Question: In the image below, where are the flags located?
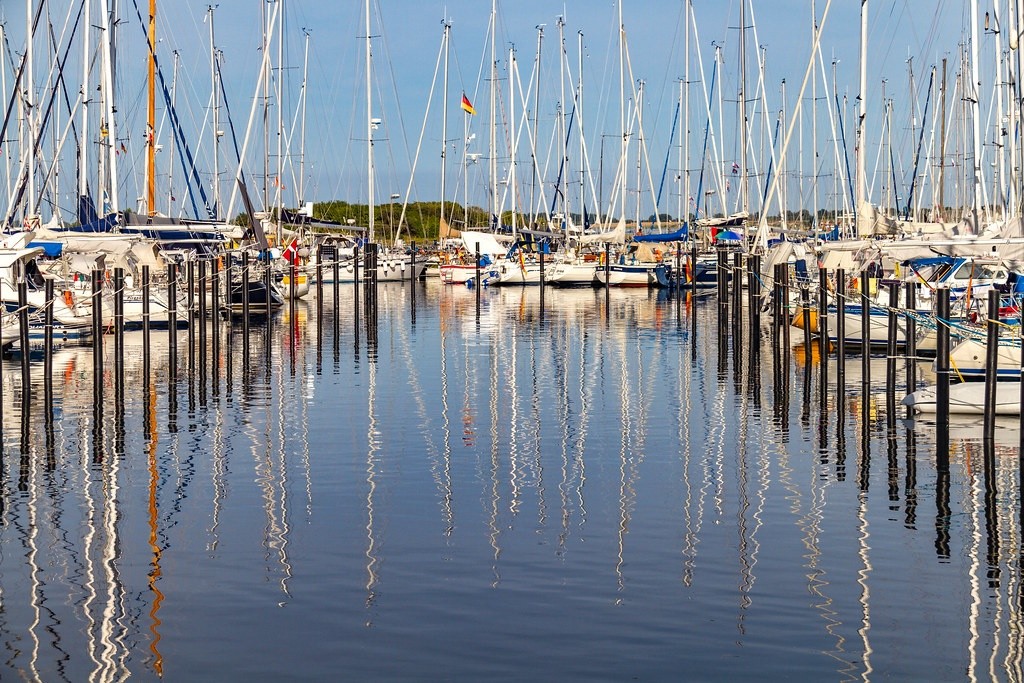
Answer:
[461,92,477,116]
[282,237,300,266]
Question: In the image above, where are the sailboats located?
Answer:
[0,1,1024,427]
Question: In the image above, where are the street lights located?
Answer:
[704,190,716,218]
[390,194,400,254]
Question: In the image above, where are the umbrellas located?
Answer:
[714,230,744,240]
